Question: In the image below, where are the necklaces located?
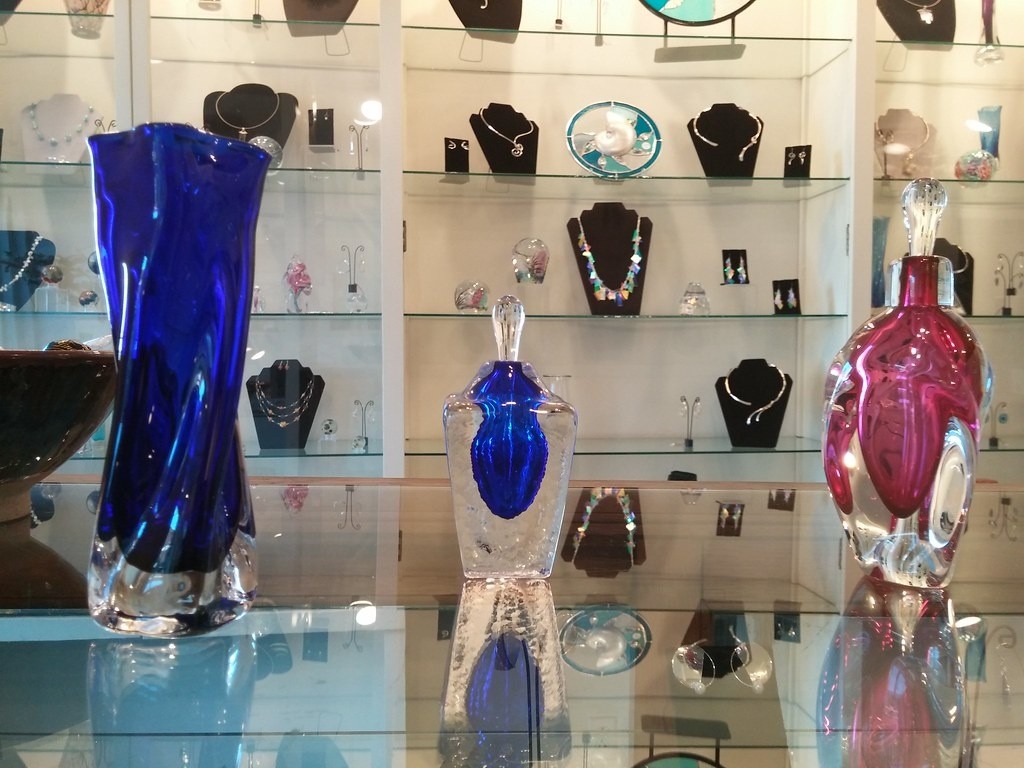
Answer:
[726,365,787,424]
[906,1,941,24]
[0,234,45,293]
[730,624,773,687]
[694,108,762,162]
[255,376,314,427]
[672,639,715,690]
[574,484,637,569]
[479,105,534,156]
[215,88,280,142]
[578,214,643,304]
[952,244,969,274]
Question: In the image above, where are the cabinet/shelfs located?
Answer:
[0,479,1024,767]
[0,2,1023,479]
